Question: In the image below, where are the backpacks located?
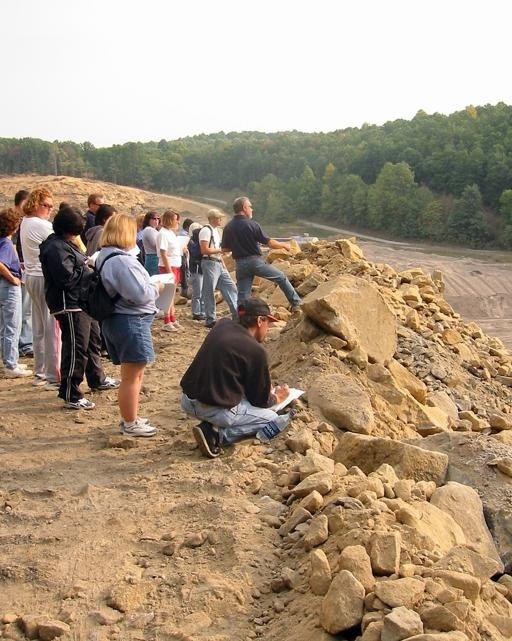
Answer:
[187,226,216,261]
[76,252,131,321]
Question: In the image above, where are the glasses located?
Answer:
[151,217,160,220]
[39,203,53,209]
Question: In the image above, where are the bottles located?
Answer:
[256,410,296,443]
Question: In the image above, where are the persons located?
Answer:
[0,188,192,437]
[221,196,303,316]
[199,209,238,327]
[186,222,206,320]
[179,296,290,459]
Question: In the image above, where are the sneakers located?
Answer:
[161,320,181,332]
[5,364,60,390]
[193,421,223,458]
[192,314,216,327]
[92,377,122,391]
[63,398,95,410]
[119,416,157,437]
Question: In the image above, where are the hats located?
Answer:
[238,296,278,322]
[207,209,226,218]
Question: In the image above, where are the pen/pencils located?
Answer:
[275,381,283,388]
